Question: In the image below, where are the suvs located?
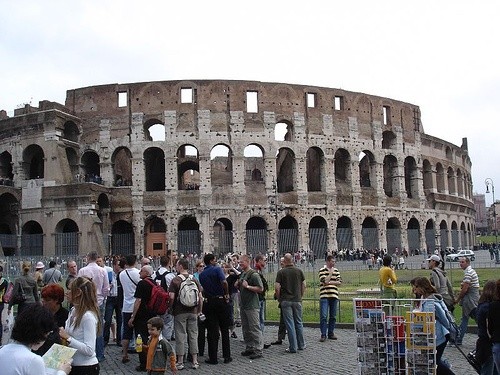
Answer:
[447,250,476,262]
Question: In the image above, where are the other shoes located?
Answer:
[270,340,283,345]
[122,357,130,363]
[104,341,109,347]
[224,357,233,363]
[285,347,292,353]
[241,350,259,359]
[205,359,218,365]
[328,333,338,340]
[446,339,463,347]
[191,362,199,369]
[117,341,122,347]
[320,335,326,342]
[231,332,237,338]
[135,366,144,372]
[199,350,204,356]
[98,355,105,362]
[176,364,185,370]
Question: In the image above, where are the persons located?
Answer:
[0,240,500,375]
[185,184,200,191]
[0,176,40,186]
[75,171,132,187]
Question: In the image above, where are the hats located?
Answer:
[425,255,440,263]
[36,262,45,269]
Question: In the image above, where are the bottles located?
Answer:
[199,313,206,321]
[136,334,142,352]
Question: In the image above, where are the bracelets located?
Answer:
[246,285,248,289]
[329,281,330,284]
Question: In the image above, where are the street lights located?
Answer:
[485,178,500,264]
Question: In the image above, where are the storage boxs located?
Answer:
[355,301,435,375]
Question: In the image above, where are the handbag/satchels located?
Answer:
[442,294,454,307]
[13,278,27,304]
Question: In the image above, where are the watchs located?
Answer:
[67,336,72,343]
[131,314,135,318]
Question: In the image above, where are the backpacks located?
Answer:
[153,270,171,292]
[177,274,199,307]
[238,270,269,301]
[144,277,170,315]
